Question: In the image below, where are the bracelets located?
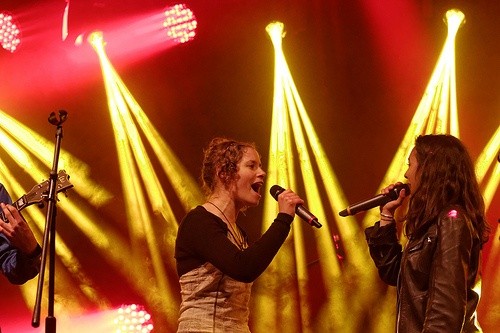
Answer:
[381,217,394,221]
[380,212,393,218]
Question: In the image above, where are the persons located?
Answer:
[363,132,492,332]
[173,136,307,332]
[1,181,44,286]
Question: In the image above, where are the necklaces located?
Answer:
[206,200,244,251]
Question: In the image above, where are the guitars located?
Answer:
[0,169,74,227]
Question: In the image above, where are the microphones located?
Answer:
[339,184,410,216]
[270,185,322,228]
